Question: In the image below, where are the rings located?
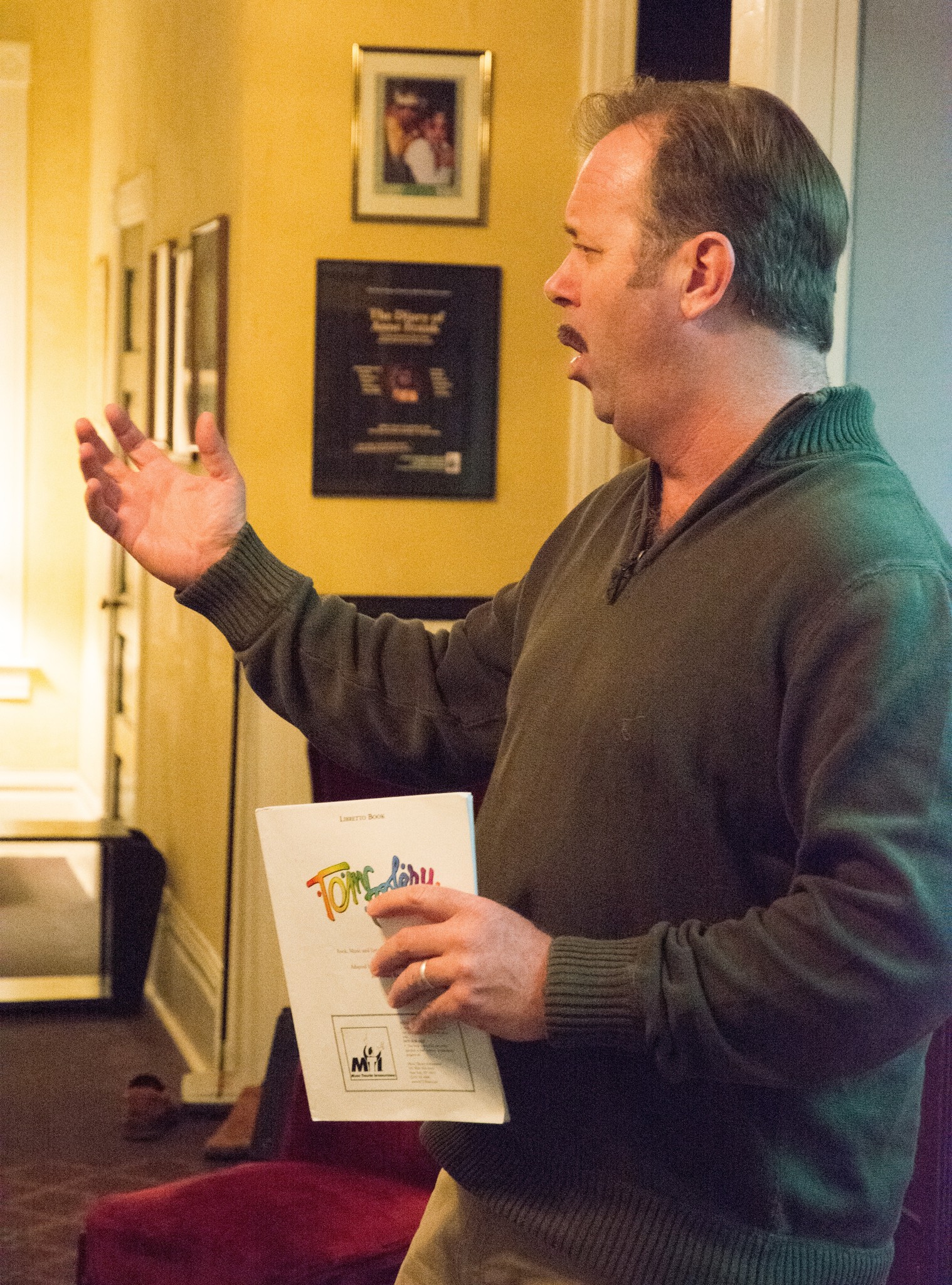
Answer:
[418,958,436,992]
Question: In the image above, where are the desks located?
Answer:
[0,814,138,1017]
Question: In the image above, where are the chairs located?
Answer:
[76,1006,440,1285]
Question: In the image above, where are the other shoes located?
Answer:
[120,1071,180,1148]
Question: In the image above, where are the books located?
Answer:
[253,788,513,1124]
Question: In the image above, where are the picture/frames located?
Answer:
[347,38,498,228]
[143,210,233,464]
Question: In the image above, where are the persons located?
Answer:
[406,114,455,188]
[384,79,437,184]
[66,79,952,1285]
